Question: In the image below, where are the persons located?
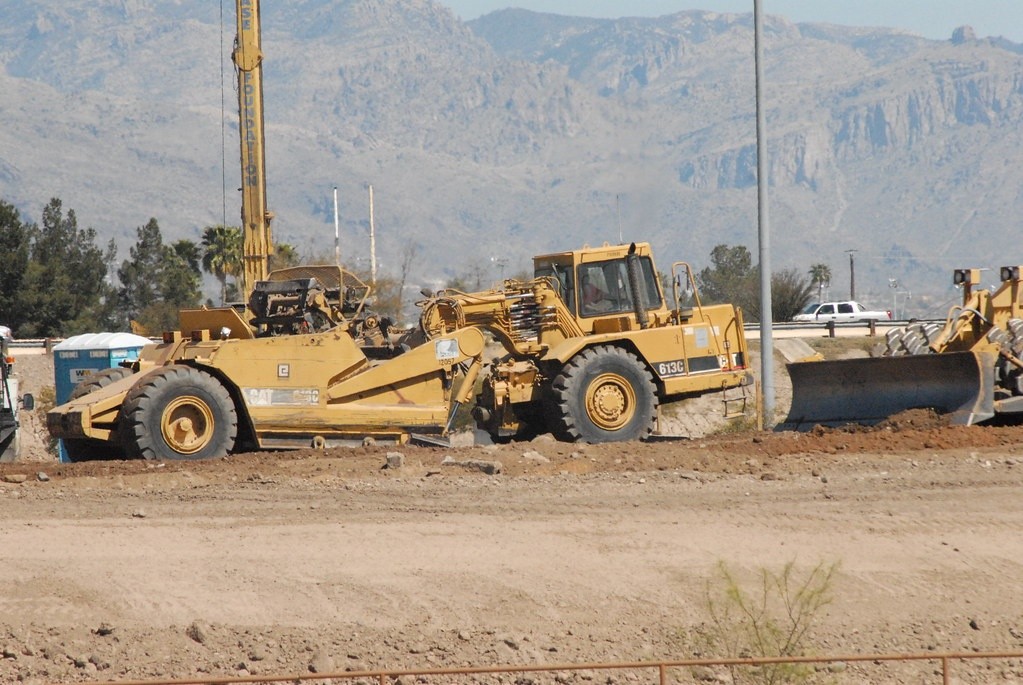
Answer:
[527,268,617,306]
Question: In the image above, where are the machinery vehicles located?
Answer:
[47,0,488,464]
[358,242,755,448]
[773,263,1023,433]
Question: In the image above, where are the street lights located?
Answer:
[845,248,858,301]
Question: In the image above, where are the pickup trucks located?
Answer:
[793,300,892,325]
